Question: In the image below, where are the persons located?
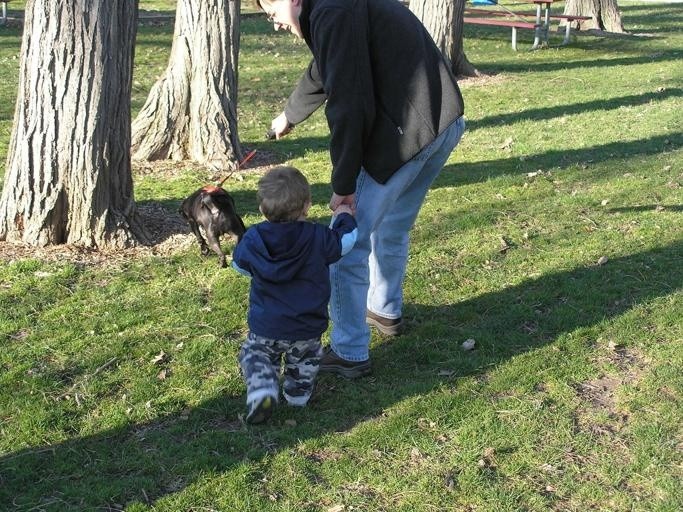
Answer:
[229,166,357,425]
[252,0,468,379]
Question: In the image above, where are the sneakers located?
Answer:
[319,345,373,378]
[289,401,307,409]
[366,309,404,336]
[246,395,272,423]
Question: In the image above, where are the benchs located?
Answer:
[462,8,594,52]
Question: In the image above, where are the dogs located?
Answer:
[177,184,248,270]
